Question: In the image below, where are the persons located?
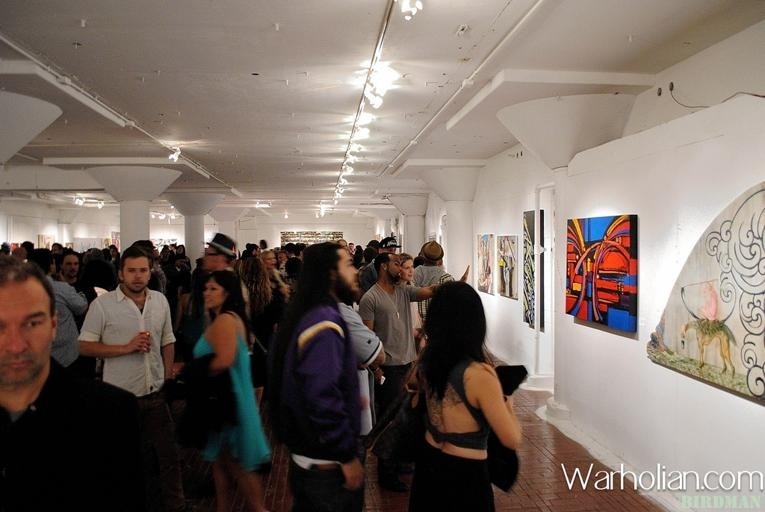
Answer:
[0,234,530,511]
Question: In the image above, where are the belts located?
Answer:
[363,365,420,470]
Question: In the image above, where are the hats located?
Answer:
[421,240,444,262]
[378,236,401,249]
[206,232,237,259]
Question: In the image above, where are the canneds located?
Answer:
[139,330,151,354]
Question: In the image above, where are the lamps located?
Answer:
[0,0,425,221]
